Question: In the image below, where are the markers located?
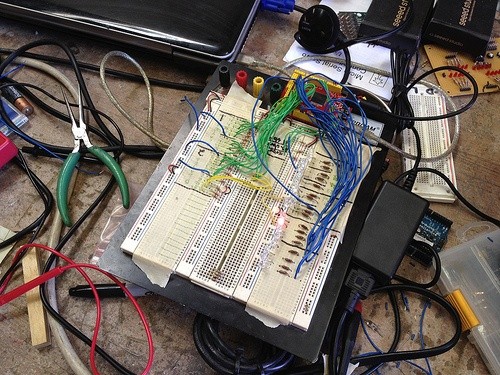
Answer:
[69,284,165,298]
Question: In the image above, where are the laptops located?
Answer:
[3,0,262,70]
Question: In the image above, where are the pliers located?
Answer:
[56,79,130,226]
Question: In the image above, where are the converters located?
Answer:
[350,180,431,282]
